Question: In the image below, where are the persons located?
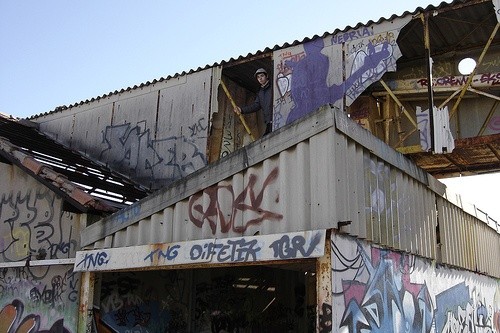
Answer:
[234,67,275,137]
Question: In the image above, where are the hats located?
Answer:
[254,68,267,77]
